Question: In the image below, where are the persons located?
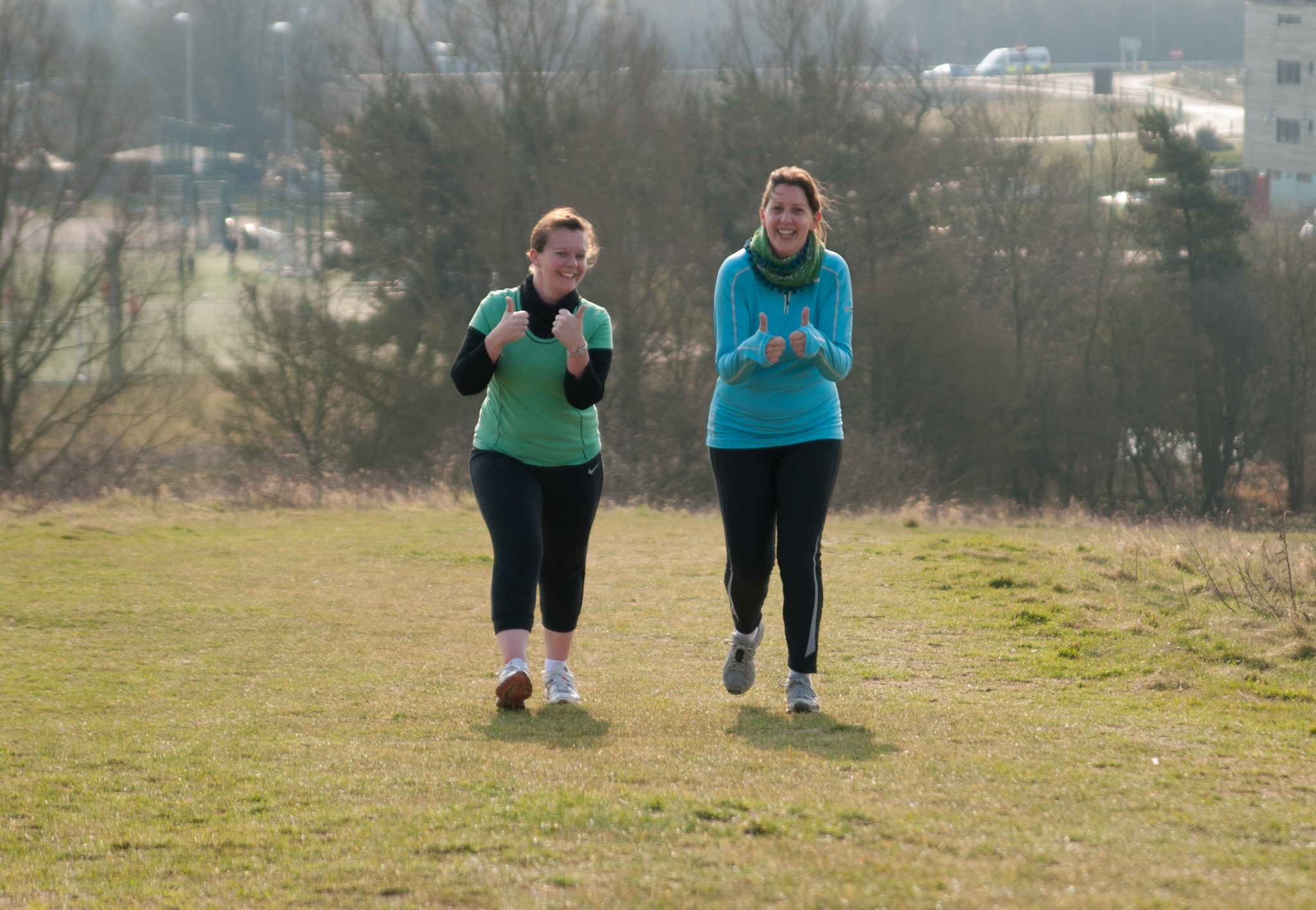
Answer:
[707,165,853,714]
[450,206,613,708]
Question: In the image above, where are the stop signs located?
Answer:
[1170,46,1184,61]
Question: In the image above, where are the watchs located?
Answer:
[567,341,588,356]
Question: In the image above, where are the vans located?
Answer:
[972,46,1051,76]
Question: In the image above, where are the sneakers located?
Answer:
[785,679,821,714]
[724,620,764,695]
[496,658,533,710]
[542,670,580,706]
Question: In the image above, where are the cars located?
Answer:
[920,64,970,78]
